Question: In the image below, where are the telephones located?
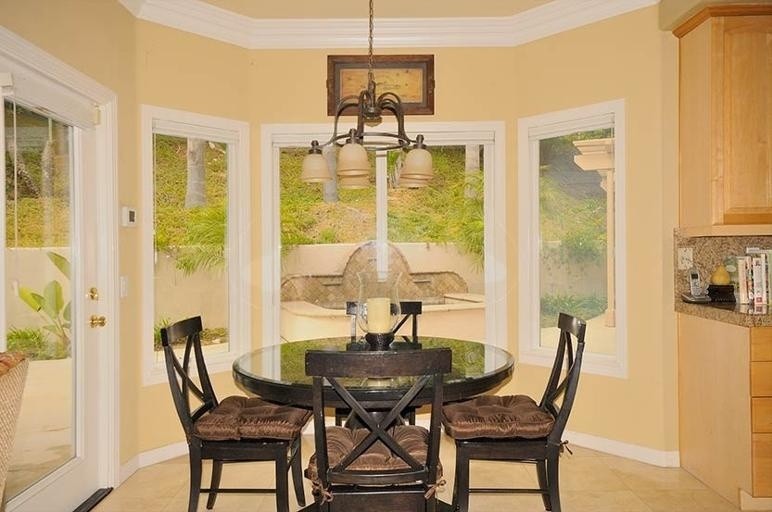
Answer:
[681,270,712,304]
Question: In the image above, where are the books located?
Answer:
[737,246,772,306]
[739,302,772,316]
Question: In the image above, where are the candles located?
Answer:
[366,297,392,333]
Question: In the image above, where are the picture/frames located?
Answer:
[326,54,435,116]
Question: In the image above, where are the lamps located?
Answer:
[297,0,435,192]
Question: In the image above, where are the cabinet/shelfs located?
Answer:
[674,8,772,227]
[679,314,772,512]
